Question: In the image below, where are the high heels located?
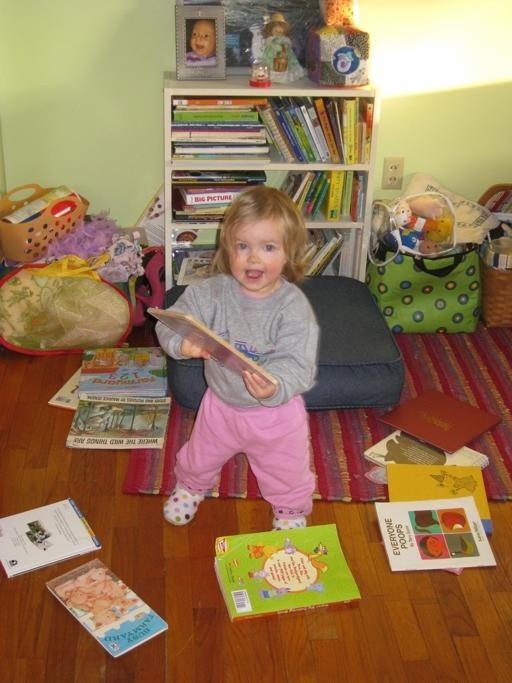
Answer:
[136,244,165,308]
[133,284,148,327]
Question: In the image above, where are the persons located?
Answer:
[247,11,305,84]
[151,185,321,533]
[185,20,217,68]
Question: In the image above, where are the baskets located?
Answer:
[481,257,512,328]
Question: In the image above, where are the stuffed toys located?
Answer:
[392,195,456,257]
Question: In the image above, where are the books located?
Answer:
[358,418,494,482]
[49,341,171,464]
[299,229,362,280]
[197,520,364,625]
[375,498,500,573]
[1,495,105,578]
[376,383,504,454]
[276,170,365,224]
[173,240,218,287]
[172,96,374,163]
[383,460,495,538]
[175,164,268,223]
[148,308,284,390]
[42,554,172,660]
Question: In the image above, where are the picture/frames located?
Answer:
[174,4,226,79]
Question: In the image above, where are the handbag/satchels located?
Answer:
[400,172,501,244]
[365,243,479,333]
[0,183,90,262]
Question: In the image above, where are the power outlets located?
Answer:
[382,157,404,188]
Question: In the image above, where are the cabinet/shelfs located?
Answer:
[163,70,382,293]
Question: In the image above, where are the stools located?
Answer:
[158,273,404,411]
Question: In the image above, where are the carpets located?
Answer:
[122,316,512,503]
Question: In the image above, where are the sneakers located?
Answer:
[271,516,306,530]
[163,482,205,525]
